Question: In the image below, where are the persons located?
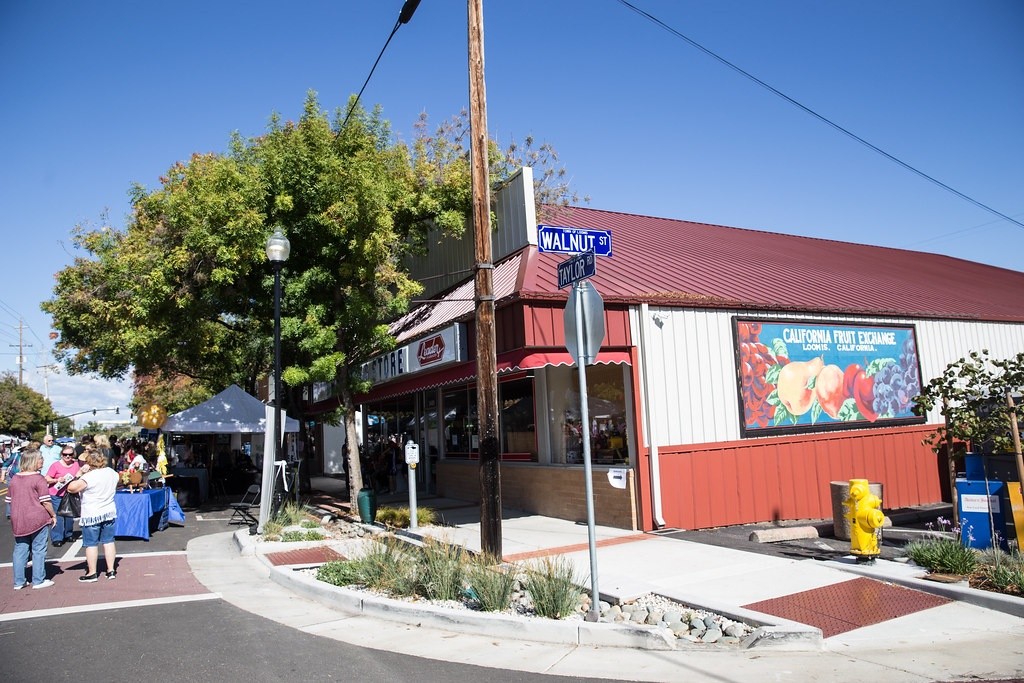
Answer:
[66,454,120,583]
[3,450,58,592]
[0,420,628,534]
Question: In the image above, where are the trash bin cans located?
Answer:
[357,488,375,523]
[954,475,1005,549]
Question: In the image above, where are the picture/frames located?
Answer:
[731,314,929,440]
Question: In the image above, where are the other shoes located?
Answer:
[65,536,73,542]
[104,570,115,579]
[78,573,98,582]
[52,540,62,547]
[13,581,30,589]
[32,579,54,589]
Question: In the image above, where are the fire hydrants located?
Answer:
[841,478,888,562]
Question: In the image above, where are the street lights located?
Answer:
[266,225,290,516]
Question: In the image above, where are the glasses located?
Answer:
[47,439,55,442]
[62,452,75,457]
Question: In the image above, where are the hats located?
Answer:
[109,434,117,441]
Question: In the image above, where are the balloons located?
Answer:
[138,402,166,430]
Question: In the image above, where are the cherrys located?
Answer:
[736,322,789,428]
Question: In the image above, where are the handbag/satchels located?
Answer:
[1,452,18,476]
[56,487,81,518]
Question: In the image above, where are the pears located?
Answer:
[776,354,846,420]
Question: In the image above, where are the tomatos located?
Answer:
[841,363,881,422]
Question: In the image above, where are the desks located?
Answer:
[114,487,170,538]
[234,467,262,501]
[173,468,208,515]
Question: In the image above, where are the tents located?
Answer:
[0,384,300,506]
[406,386,618,463]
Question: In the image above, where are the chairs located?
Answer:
[274,469,295,515]
[228,485,260,526]
